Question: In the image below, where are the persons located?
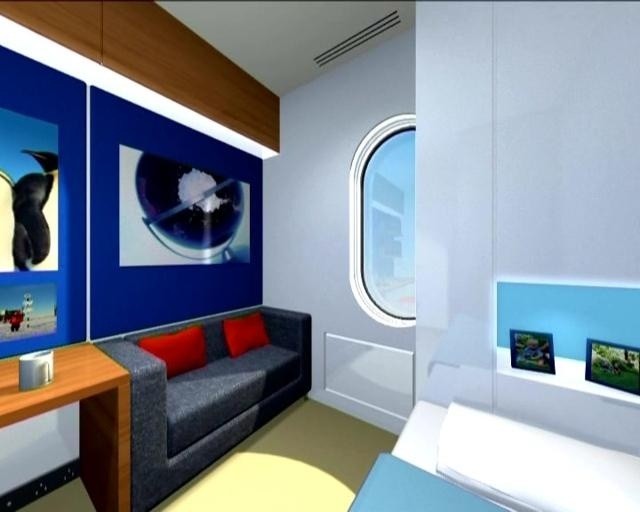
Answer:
[539,343,549,360]
[8,311,23,334]
[517,339,546,367]
[599,359,622,377]
[514,335,526,350]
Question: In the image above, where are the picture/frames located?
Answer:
[583,337,640,397]
[508,328,556,375]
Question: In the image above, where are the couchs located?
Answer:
[94,305,312,512]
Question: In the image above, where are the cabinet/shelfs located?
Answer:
[0,1,281,160]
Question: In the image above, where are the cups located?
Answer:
[19,348,54,389]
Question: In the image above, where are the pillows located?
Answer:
[219,312,270,360]
[137,326,209,382]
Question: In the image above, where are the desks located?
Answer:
[1,342,131,512]
